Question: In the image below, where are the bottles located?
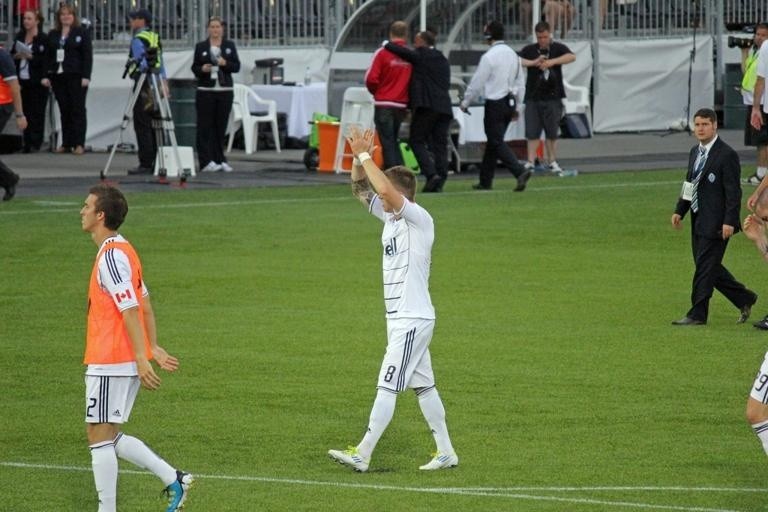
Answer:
[304,67,311,87]
[559,170,578,177]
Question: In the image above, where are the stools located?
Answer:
[153,146,196,178]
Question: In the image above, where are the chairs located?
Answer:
[226,83,282,155]
[79,0,346,41]
[562,77,593,135]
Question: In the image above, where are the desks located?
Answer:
[450,103,562,163]
[246,83,327,140]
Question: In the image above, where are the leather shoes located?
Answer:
[128,164,152,174]
[471,183,492,188]
[422,173,445,191]
[515,170,532,191]
[672,316,707,325]
[737,292,757,324]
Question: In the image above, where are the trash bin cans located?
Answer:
[724,63,747,129]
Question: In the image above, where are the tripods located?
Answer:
[99,73,187,184]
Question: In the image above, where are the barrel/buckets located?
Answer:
[721,63,746,130]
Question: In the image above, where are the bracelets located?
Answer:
[358,152,371,163]
[758,243,768,256]
[352,157,361,167]
[15,112,24,118]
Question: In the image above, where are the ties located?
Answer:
[691,147,706,212]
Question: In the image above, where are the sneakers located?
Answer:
[202,162,221,172]
[548,162,561,173]
[524,162,536,172]
[419,450,458,470]
[160,470,193,511]
[328,445,370,472]
[221,163,233,173]
[754,315,768,330]
[740,173,765,185]
[4,174,19,202]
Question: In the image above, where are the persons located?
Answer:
[382,32,452,193]
[125,9,168,176]
[459,21,531,193]
[9,9,49,154]
[79,187,192,512]
[0,47,28,203]
[740,23,768,184]
[672,108,757,325]
[743,214,768,456]
[329,124,458,474]
[750,40,768,131]
[189,16,240,174]
[742,169,768,211]
[41,3,93,156]
[365,21,414,171]
[518,22,576,176]
